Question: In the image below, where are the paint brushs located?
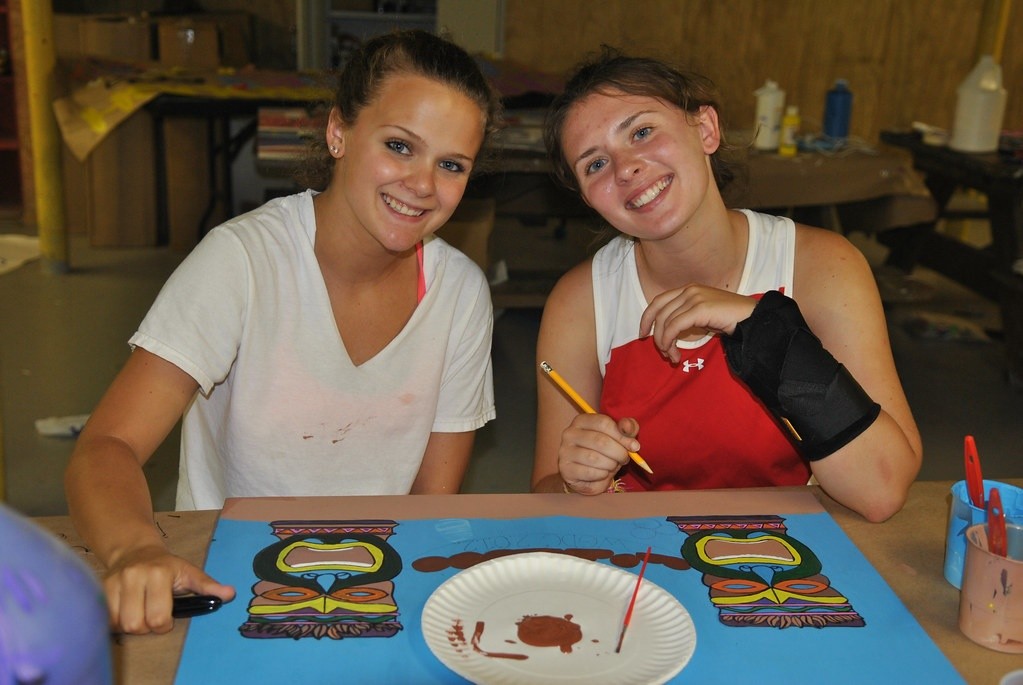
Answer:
[616,546,652,654]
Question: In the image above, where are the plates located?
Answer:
[421,552,696,685]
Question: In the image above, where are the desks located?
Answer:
[474,134,914,310]
[25,474,1023,685]
[153,96,332,239]
[881,131,1023,388]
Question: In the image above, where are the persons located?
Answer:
[70,28,501,637]
[531,52,925,525]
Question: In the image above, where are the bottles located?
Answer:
[752,80,785,149]
[823,82,852,138]
[948,54,1009,152]
[778,105,801,157]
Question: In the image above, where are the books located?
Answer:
[256,105,332,164]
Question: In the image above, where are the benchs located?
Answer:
[874,197,1023,396]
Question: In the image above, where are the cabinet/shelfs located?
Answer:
[297,0,435,112]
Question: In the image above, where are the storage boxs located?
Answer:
[52,12,258,250]
[440,197,496,275]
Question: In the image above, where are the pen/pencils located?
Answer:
[540,361,656,476]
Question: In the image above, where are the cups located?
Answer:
[958,523,1023,654]
[943,479,1023,591]
